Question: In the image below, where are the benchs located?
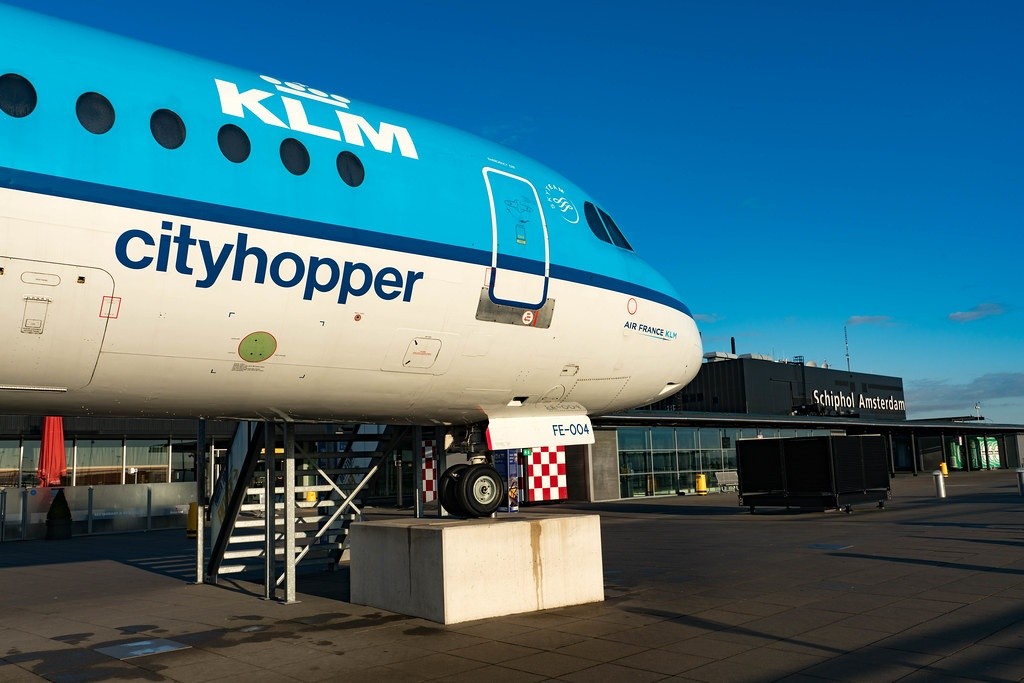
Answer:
[715,471,739,494]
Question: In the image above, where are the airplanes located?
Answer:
[1,0,703,519]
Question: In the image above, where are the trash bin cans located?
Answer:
[696,473,707,496]
[939,461,949,477]
[647,475,658,496]
